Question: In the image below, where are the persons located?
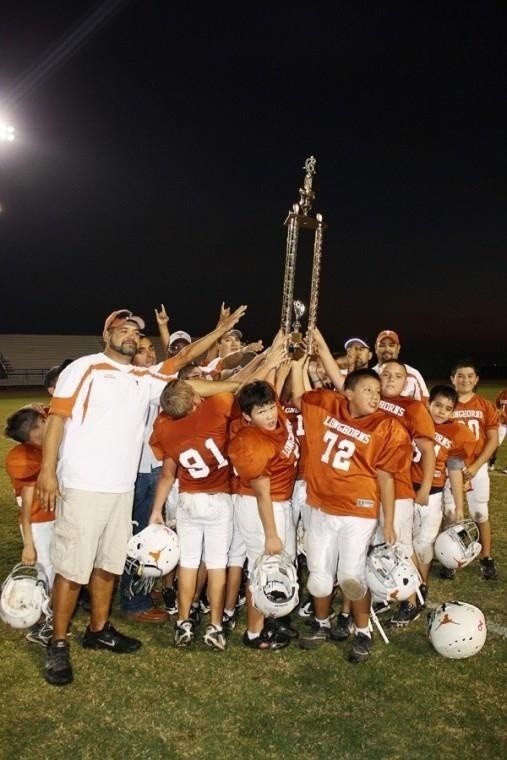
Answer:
[4,302,505,687]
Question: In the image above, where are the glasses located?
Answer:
[107,311,141,330]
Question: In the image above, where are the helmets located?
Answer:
[248,553,300,619]
[124,523,181,577]
[0,562,50,629]
[366,544,424,603]
[434,519,483,570]
[428,600,487,660]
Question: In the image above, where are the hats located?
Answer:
[377,330,399,344]
[102,309,145,340]
[168,331,192,354]
[216,329,243,342]
[344,338,370,350]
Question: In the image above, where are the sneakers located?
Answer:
[120,584,247,652]
[243,598,372,663]
[479,556,499,580]
[440,566,456,579]
[77,588,111,618]
[374,584,426,628]
[25,623,73,685]
[82,620,142,653]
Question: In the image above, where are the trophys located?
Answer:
[278,155,331,369]
